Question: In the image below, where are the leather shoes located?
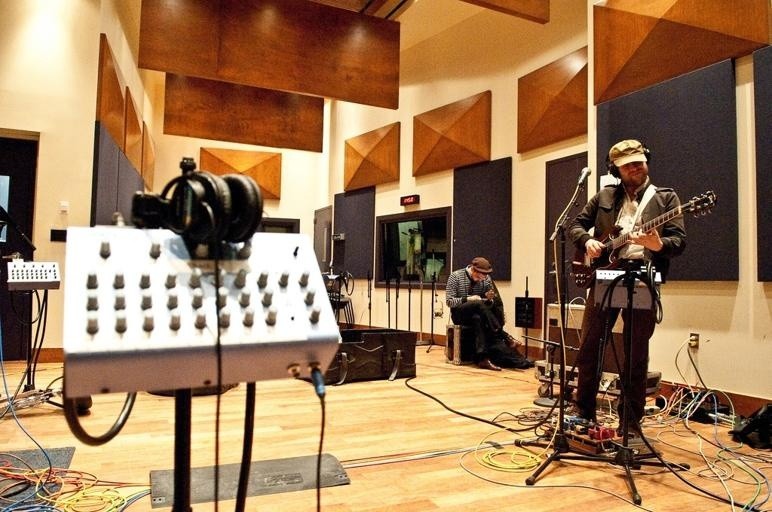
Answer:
[479,359,502,371]
[506,332,521,349]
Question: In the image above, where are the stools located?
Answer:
[446,324,482,365]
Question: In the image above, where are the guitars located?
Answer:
[572,190,719,289]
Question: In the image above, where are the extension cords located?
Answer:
[549,416,621,455]
[674,387,719,407]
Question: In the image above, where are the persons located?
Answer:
[446,256,516,371]
[569,139,686,438]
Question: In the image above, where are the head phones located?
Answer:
[606,142,652,176]
[128,172,264,243]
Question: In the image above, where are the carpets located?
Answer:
[0,447,77,512]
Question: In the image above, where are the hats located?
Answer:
[472,257,493,274]
[609,140,647,167]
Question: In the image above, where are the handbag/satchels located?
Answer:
[488,339,533,369]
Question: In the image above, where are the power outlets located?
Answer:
[690,333,699,348]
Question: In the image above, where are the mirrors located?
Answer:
[374,206,452,289]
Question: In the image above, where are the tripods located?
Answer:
[0,299,63,410]
[513,226,642,485]
[419,276,447,352]
[549,267,691,504]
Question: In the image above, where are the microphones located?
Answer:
[576,166,592,190]
